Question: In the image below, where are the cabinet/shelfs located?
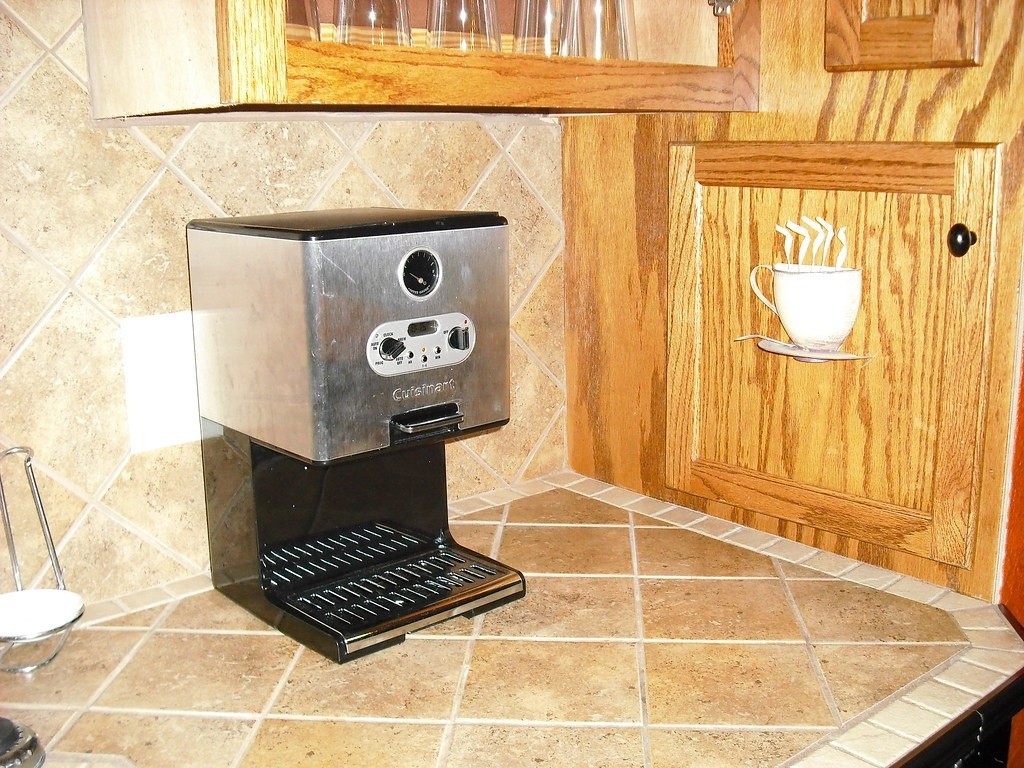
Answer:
[80,0,763,131]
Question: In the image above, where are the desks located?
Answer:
[0,472,1024,768]
